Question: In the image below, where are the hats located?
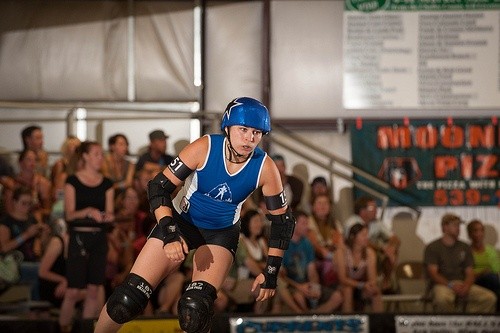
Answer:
[149,129,169,142]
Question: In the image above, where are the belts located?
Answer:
[74,227,100,232]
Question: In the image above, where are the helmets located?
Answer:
[221,96,271,137]
